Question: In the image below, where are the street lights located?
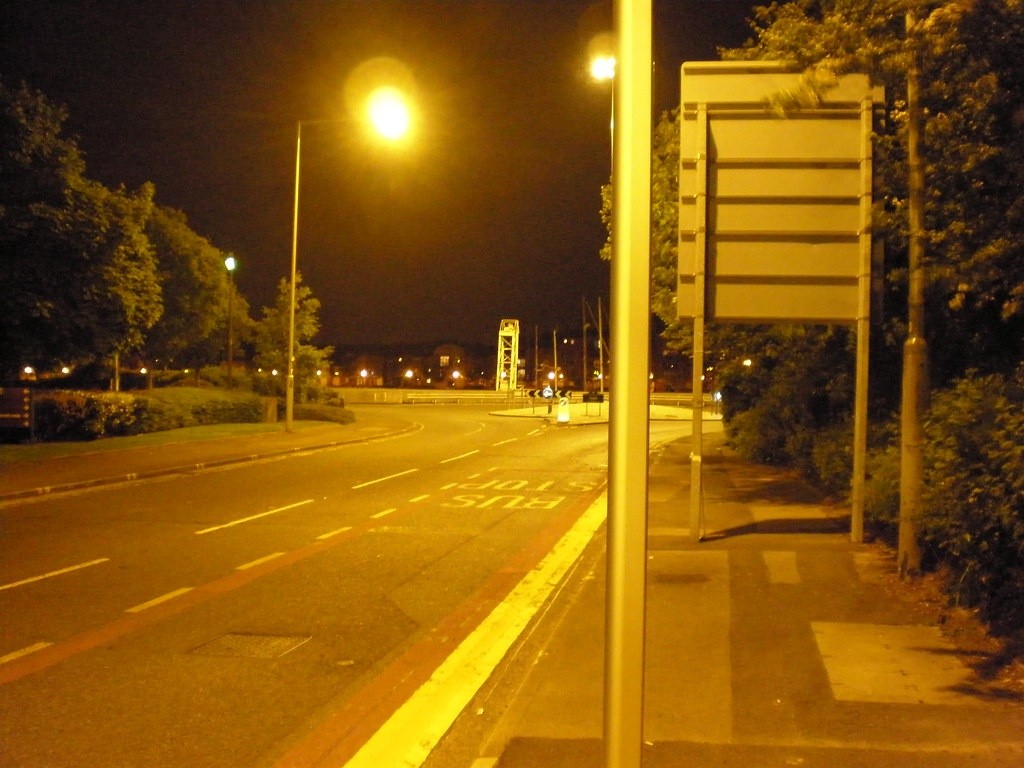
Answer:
[223,256,237,391]
[283,91,413,434]
[591,55,614,176]
